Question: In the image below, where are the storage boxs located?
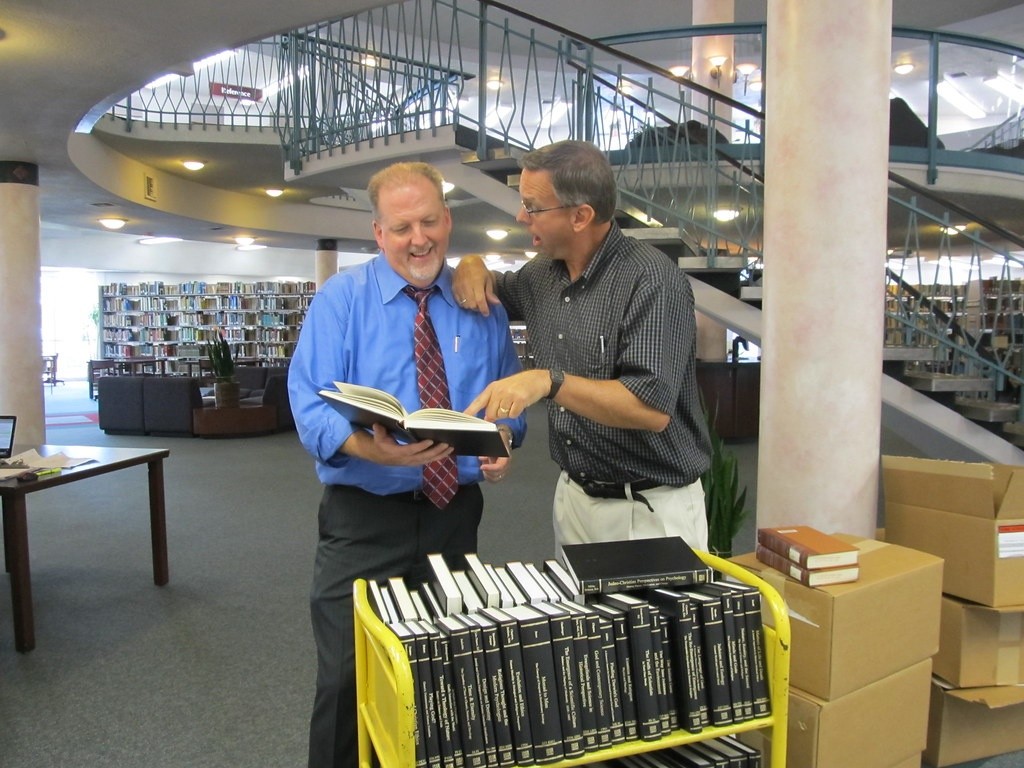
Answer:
[724,455,1024,768]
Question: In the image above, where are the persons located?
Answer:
[452,141,713,554]
[286,161,529,768]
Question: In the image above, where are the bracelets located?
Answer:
[497,426,512,445]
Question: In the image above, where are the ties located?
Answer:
[402,285,460,511]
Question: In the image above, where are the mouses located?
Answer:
[17,471,38,482]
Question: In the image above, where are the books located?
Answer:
[886,280,1024,346]
[314,380,510,458]
[757,525,859,587]
[102,280,315,359]
[363,536,773,768]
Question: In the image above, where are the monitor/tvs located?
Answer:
[0,415,17,459]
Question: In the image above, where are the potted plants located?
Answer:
[205,330,242,409]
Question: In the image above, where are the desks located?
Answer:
[193,404,278,438]
[86,358,167,400]
[184,359,264,378]
[0,444,171,653]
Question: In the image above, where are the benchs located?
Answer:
[97,367,295,437]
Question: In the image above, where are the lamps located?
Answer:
[733,62,761,96]
[669,66,693,82]
[709,55,729,88]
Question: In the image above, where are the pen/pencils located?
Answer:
[600,336,604,353]
[455,334,460,353]
[36,468,61,476]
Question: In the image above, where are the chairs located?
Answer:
[199,359,215,387]
[89,355,157,400]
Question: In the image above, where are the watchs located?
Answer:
[546,369,564,399]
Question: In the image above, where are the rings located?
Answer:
[499,475,502,479]
[460,298,466,304]
[501,408,510,414]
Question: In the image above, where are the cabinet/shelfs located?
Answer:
[884,280,1024,372]
[352,550,791,768]
[100,281,317,377]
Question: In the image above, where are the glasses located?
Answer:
[520,201,574,218]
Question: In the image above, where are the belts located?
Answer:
[567,473,664,512]
[401,491,429,505]
